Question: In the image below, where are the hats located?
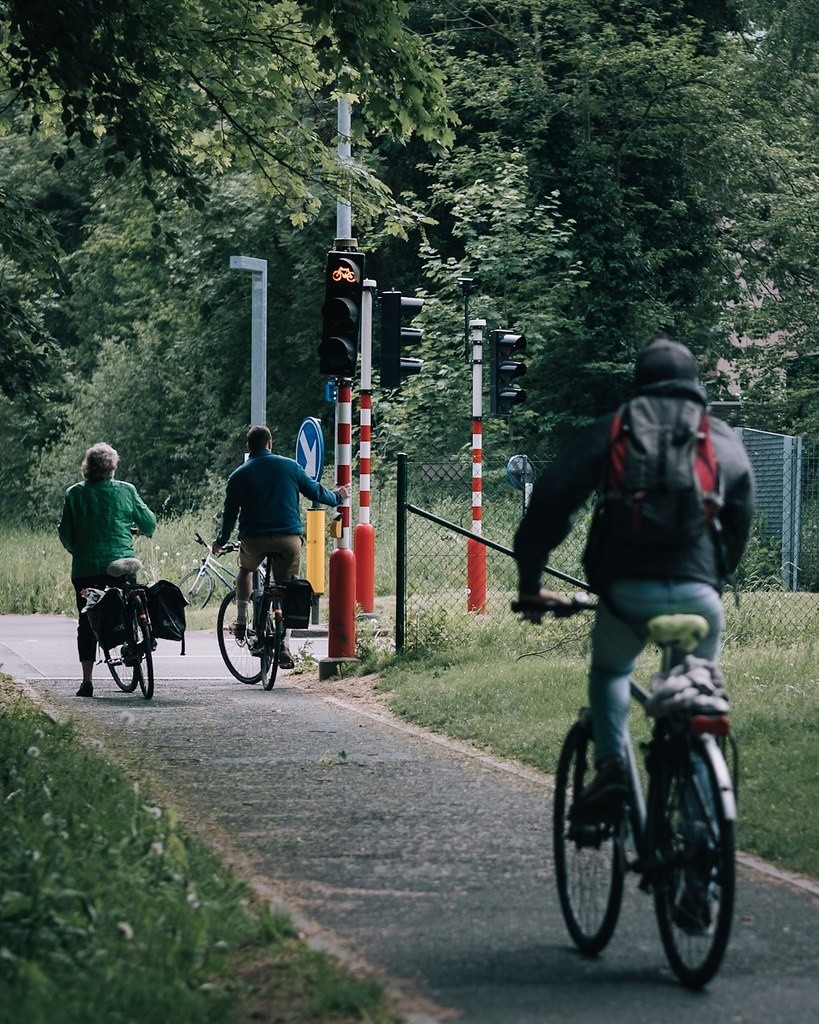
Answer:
[634,336,700,382]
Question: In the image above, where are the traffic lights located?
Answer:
[376,287,427,389]
[488,325,529,416]
[315,246,367,378]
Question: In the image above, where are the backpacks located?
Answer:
[596,390,727,553]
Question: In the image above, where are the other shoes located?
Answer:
[567,752,634,826]
[279,642,295,669]
[76,681,94,697]
[674,886,713,938]
[228,621,246,638]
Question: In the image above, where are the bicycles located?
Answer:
[175,531,268,610]
[90,527,159,701]
[509,592,743,990]
[215,540,291,694]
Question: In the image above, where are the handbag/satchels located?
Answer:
[279,574,316,629]
[141,579,190,641]
[85,588,130,650]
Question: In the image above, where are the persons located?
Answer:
[511,342,757,935]
[58,443,157,697]
[211,426,352,669]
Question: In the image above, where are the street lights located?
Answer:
[227,255,271,425]
